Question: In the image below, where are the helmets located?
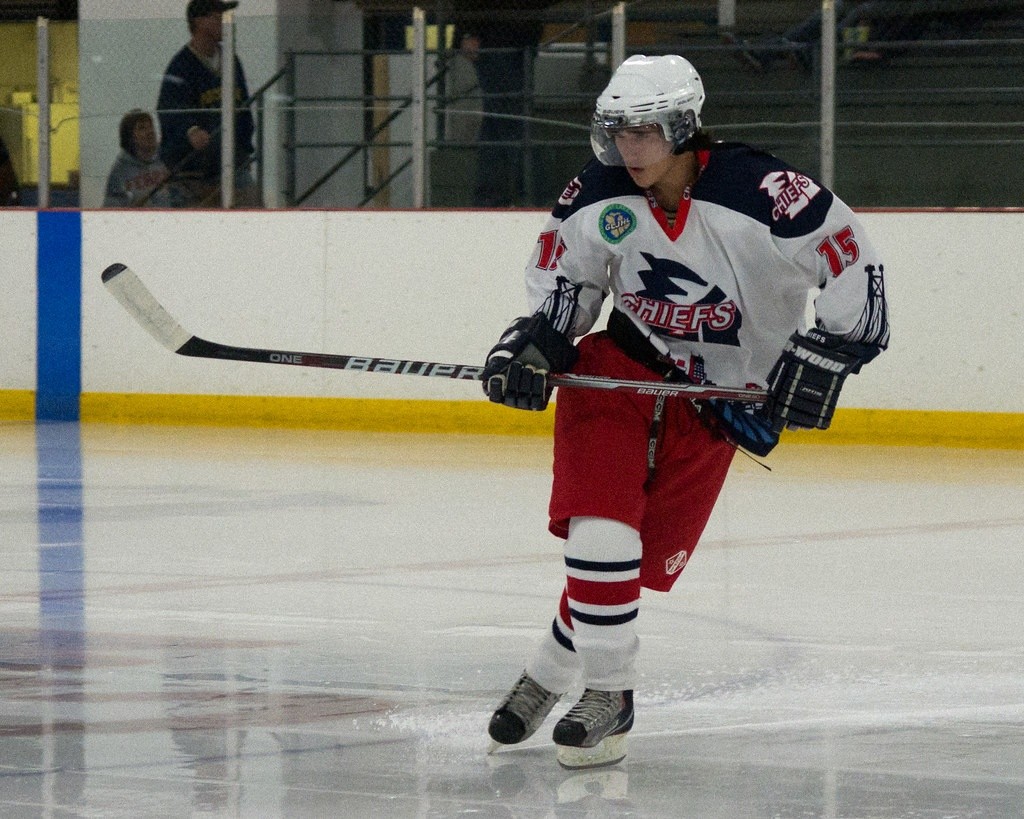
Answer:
[594,54,706,145]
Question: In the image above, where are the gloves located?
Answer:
[482,311,577,412]
[767,327,882,432]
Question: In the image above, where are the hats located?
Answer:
[186,0,239,18]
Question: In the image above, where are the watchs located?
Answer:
[7,190,20,201]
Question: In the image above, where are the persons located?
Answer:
[0,137,23,207]
[156,0,266,209]
[453,0,561,209]
[100,107,183,209]
[481,52,893,770]
[740,0,887,74]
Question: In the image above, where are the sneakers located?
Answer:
[552,688,634,748]
[488,668,564,744]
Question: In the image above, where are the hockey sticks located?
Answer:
[102,261,774,402]
